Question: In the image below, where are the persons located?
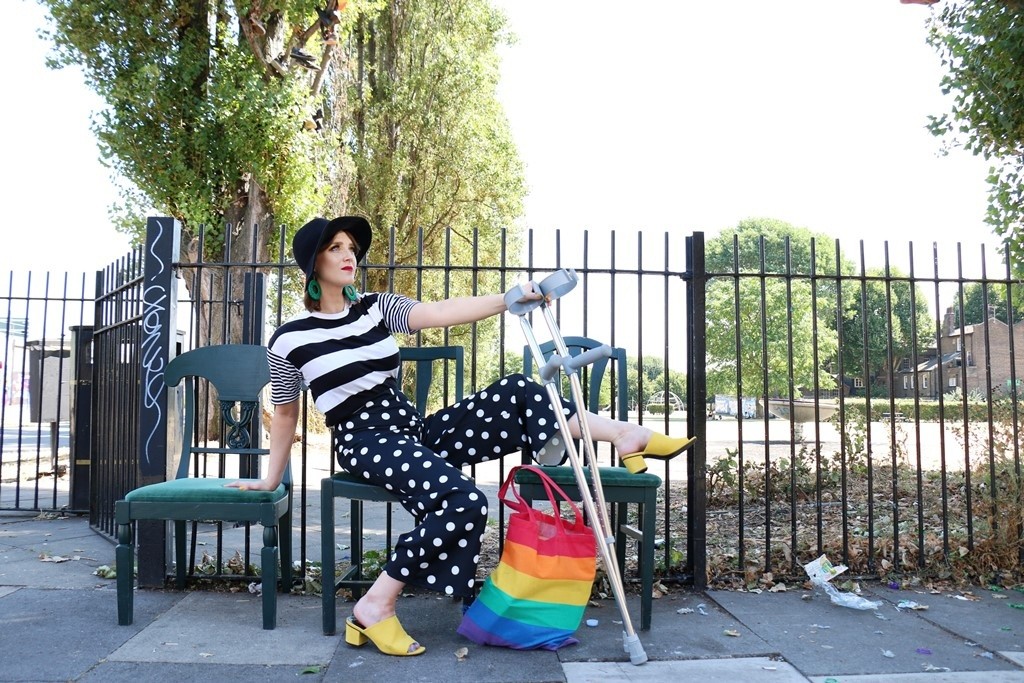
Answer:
[223,217,694,656]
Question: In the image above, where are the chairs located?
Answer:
[514,336,662,631]
[115,344,293,630]
[321,345,475,637]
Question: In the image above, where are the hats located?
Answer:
[294,216,372,278]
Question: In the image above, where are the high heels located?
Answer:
[622,432,698,475]
[346,614,427,656]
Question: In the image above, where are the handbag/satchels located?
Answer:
[456,467,598,650]
